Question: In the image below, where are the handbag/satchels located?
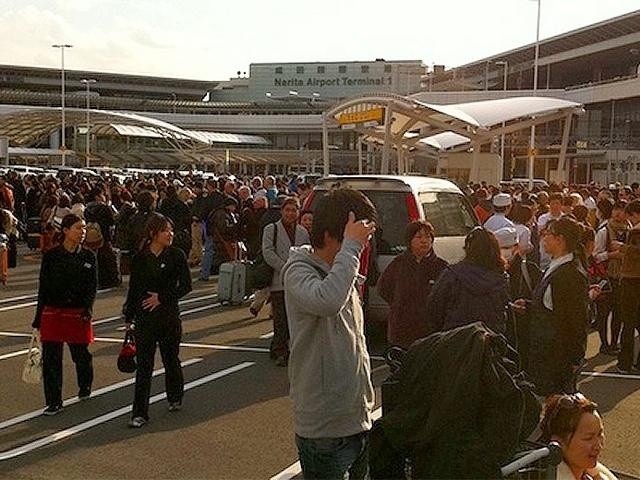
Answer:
[246,246,277,290]
[370,320,542,442]
[118,331,137,372]
[22,328,44,384]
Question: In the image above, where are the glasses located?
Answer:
[544,231,551,237]
[547,392,586,435]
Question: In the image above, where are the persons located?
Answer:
[100,170,141,213]
[251,196,274,232]
[611,198,640,376]
[280,186,376,479]
[264,170,315,215]
[594,199,614,232]
[24,170,62,252]
[356,234,381,337]
[298,209,314,236]
[462,181,480,207]
[189,183,207,267]
[206,198,239,274]
[471,189,495,226]
[376,220,450,352]
[514,218,589,395]
[218,175,240,202]
[157,186,193,260]
[32,214,99,415]
[426,227,510,335]
[549,181,576,217]
[493,227,542,300]
[84,187,122,290]
[193,174,218,201]
[542,392,619,480]
[512,206,535,260]
[510,181,529,209]
[594,200,633,357]
[263,197,311,367]
[575,181,600,206]
[141,173,168,211]
[538,194,567,271]
[529,185,548,224]
[240,174,264,208]
[0,170,25,288]
[198,179,225,282]
[482,194,516,232]
[168,173,192,208]
[480,181,510,192]
[119,192,165,269]
[600,182,640,204]
[62,169,99,221]
[572,205,595,258]
[124,213,193,428]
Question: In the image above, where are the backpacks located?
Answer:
[83,211,105,253]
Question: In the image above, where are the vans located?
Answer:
[298,174,488,328]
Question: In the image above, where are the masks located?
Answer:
[505,205,513,216]
[500,246,517,260]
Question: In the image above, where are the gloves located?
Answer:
[32,312,40,328]
[80,312,92,323]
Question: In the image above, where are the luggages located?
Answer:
[98,248,122,287]
[218,240,246,306]
[25,217,41,252]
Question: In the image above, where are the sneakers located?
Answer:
[609,344,621,355]
[79,383,91,401]
[275,355,288,366]
[632,362,638,371]
[43,400,65,416]
[168,398,184,412]
[600,346,613,357]
[616,363,630,374]
[129,415,148,429]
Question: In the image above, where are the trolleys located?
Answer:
[361,406,562,480]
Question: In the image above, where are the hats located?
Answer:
[493,193,512,206]
[173,179,184,187]
[493,227,519,248]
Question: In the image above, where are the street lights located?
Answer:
[51,43,74,168]
[494,59,509,92]
[80,79,97,169]
